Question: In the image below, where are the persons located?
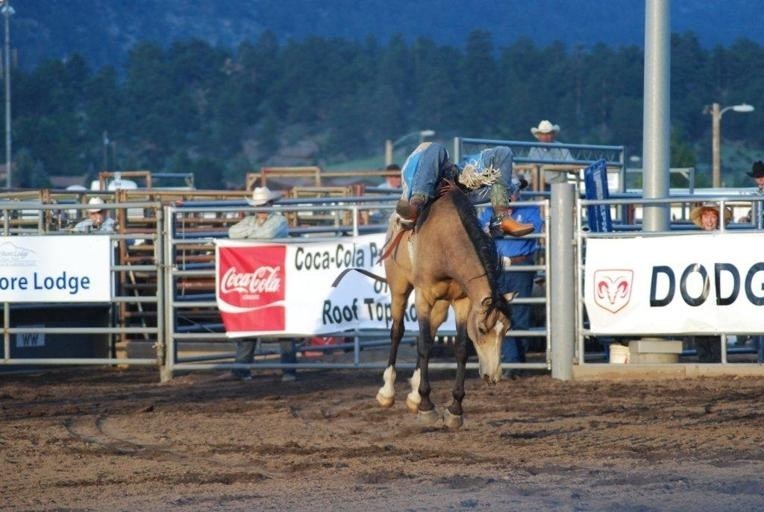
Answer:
[70,197,143,369]
[736,160,764,227]
[688,201,732,363]
[374,162,405,224]
[476,175,543,382]
[212,185,299,383]
[525,120,579,188]
[395,142,536,241]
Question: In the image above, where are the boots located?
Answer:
[396,198,425,229]
[493,205,534,238]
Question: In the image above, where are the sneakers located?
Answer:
[283,373,297,382]
[220,372,251,381]
[501,359,526,380]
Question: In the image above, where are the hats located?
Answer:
[85,197,105,213]
[530,120,560,139]
[691,202,732,226]
[379,166,401,177]
[747,161,764,177]
[516,175,528,188]
[242,186,281,206]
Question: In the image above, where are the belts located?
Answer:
[511,256,527,263]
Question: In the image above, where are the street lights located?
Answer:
[2,3,16,189]
[712,103,755,188]
[385,130,435,167]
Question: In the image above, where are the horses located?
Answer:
[375,178,520,431]
